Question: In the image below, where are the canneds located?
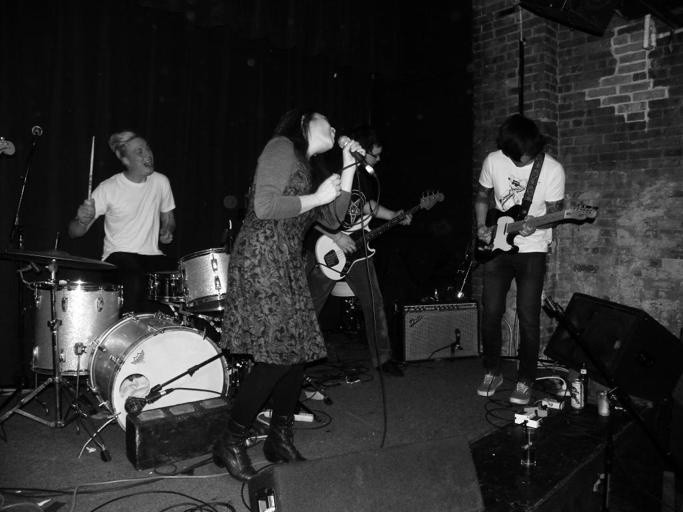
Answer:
[571,378,585,410]
[597,389,612,418]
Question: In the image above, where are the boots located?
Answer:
[264,411,307,463]
[214,417,258,481]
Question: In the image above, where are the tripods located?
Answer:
[304,374,334,407]
[0,261,117,462]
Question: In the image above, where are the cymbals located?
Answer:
[5,249,118,267]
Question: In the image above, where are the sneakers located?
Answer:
[510,383,531,404]
[380,358,404,377]
[477,374,503,396]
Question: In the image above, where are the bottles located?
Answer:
[578,361,588,404]
[434,288,439,302]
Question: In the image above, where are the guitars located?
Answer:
[315,190,444,281]
[474,204,599,264]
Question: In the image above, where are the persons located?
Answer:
[302,124,413,390]
[68,129,176,317]
[212,104,366,482]
[475,114,567,407]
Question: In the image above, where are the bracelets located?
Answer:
[334,231,342,242]
[78,220,85,226]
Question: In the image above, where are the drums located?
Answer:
[30,279,123,377]
[179,247,231,311]
[87,313,230,434]
[147,273,182,303]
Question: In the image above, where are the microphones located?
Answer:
[223,195,237,238]
[30,123,45,149]
[125,387,174,415]
[338,135,374,176]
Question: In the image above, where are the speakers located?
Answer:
[543,291,683,406]
[469,399,666,511]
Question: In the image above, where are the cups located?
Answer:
[596,390,609,417]
